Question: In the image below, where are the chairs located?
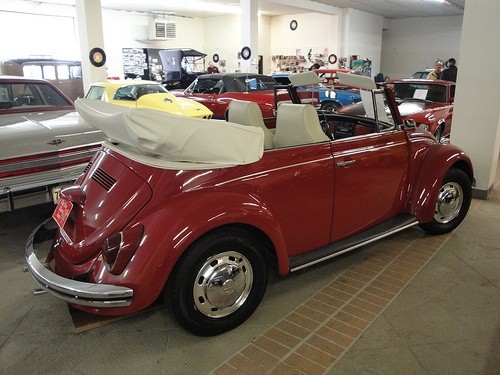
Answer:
[230,100,274,150]
[273,103,330,149]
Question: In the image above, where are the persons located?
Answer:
[426,63,442,80]
[441,58,458,80]
[207,61,219,73]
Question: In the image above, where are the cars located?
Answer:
[321,68,456,138]
[168,54,367,128]
[82,83,214,120]
[24,70,477,337]
[0,56,87,215]
